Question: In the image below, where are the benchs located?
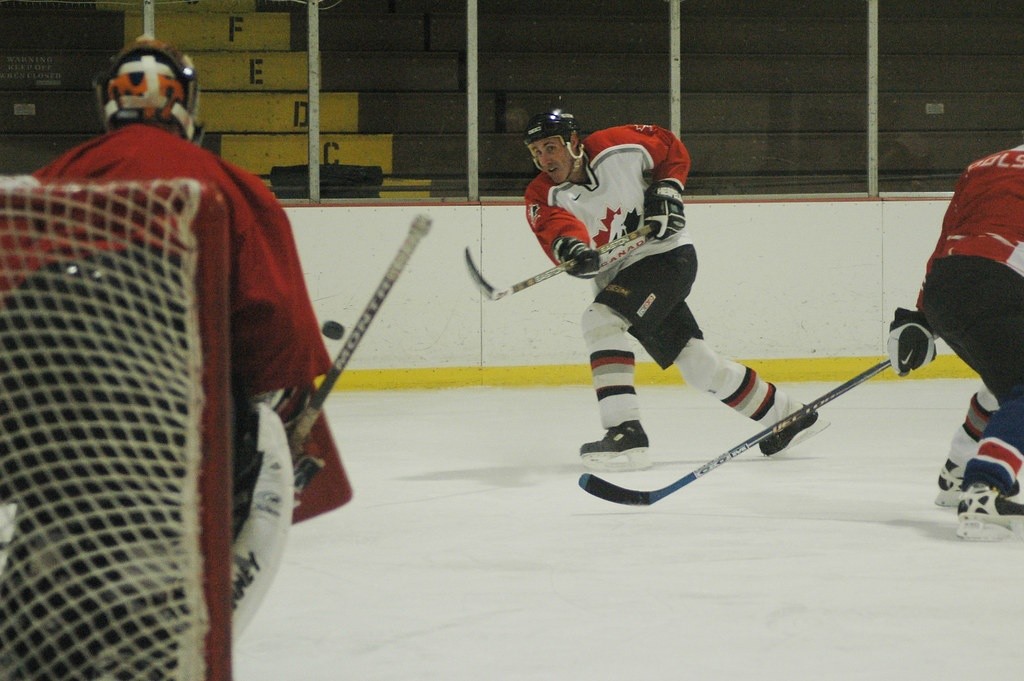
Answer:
[0,0,1024,202]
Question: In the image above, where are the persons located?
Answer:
[887,143,1024,542]
[0,34,354,681]
[524,105,832,471]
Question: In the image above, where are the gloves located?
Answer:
[550,235,600,279]
[887,306,936,377]
[644,178,686,244]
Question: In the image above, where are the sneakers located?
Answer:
[759,402,831,457]
[956,479,1024,540]
[580,420,660,473]
[935,457,966,507]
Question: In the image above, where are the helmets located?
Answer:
[522,108,579,158]
[102,47,195,138]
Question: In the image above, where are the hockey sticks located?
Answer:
[579,358,901,506]
[289,215,436,503]
[463,221,657,301]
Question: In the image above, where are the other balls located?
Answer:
[321,321,345,340]
